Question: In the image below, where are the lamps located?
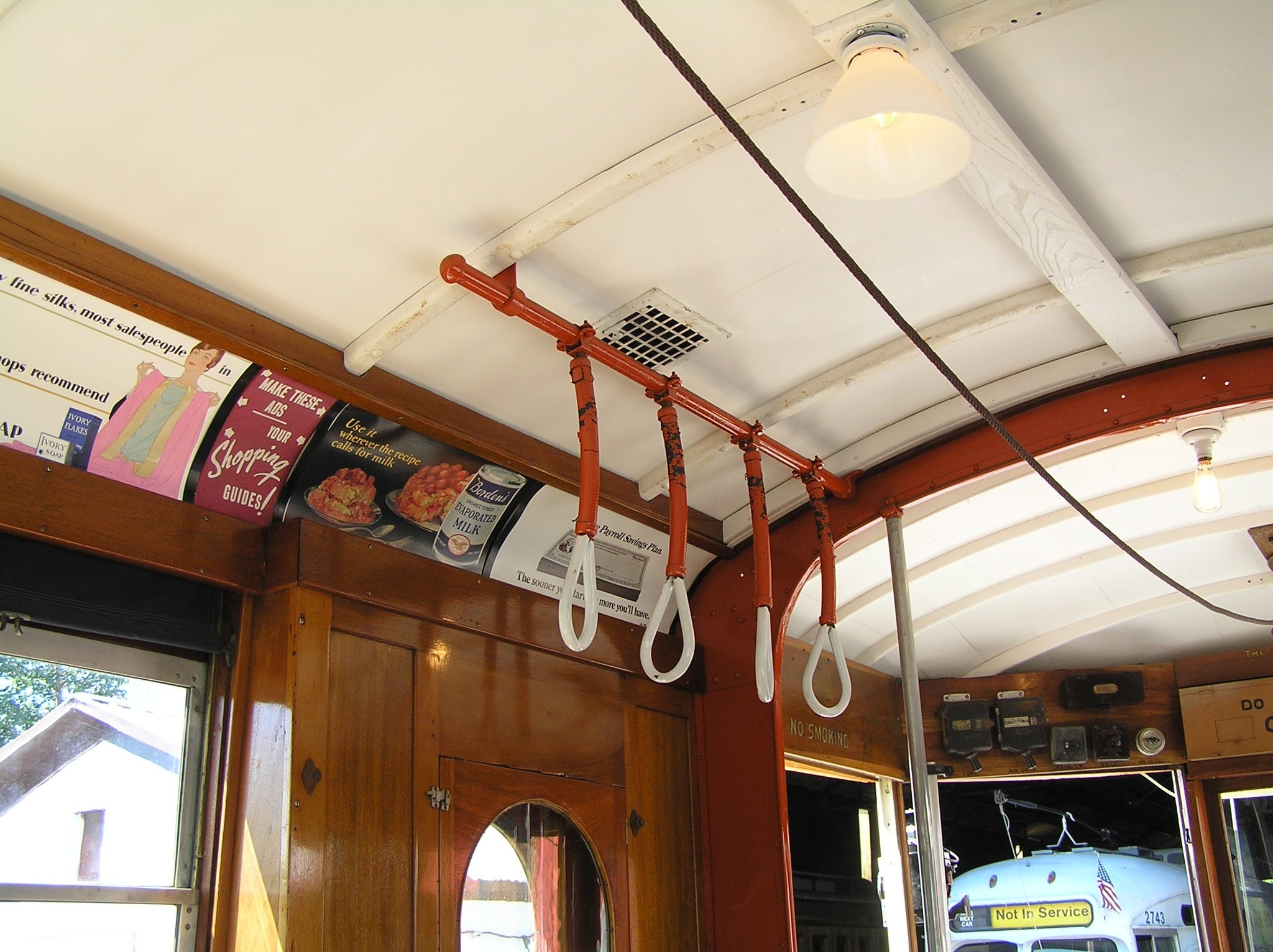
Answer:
[807,31,973,205]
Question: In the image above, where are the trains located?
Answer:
[948,845,1203,952]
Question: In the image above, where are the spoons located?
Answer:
[338,525,395,538]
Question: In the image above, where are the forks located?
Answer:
[366,535,415,548]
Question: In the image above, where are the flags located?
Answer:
[1096,861,1121,914]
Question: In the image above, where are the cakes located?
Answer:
[396,463,479,525]
[309,467,376,523]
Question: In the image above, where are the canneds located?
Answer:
[433,463,527,568]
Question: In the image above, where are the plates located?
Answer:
[303,486,383,527]
[385,489,442,534]
